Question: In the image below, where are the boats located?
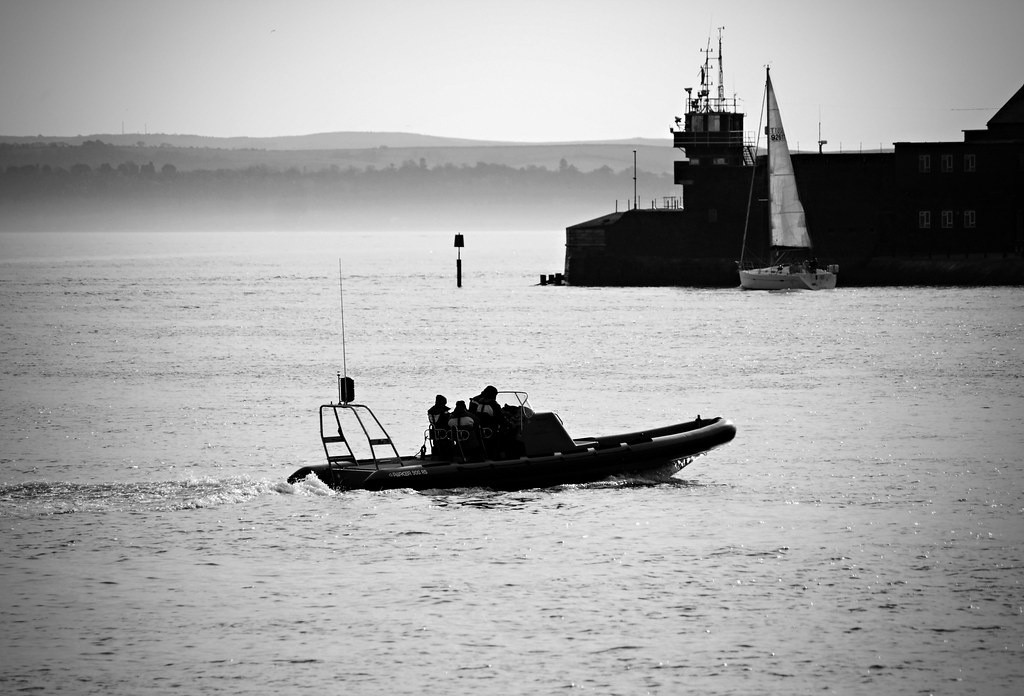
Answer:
[286,376,739,493]
[537,25,1024,288]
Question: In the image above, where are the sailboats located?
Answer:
[740,63,838,291]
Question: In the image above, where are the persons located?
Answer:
[469,386,513,458]
[809,258,819,269]
[428,395,453,438]
[778,264,783,274]
[450,400,469,418]
